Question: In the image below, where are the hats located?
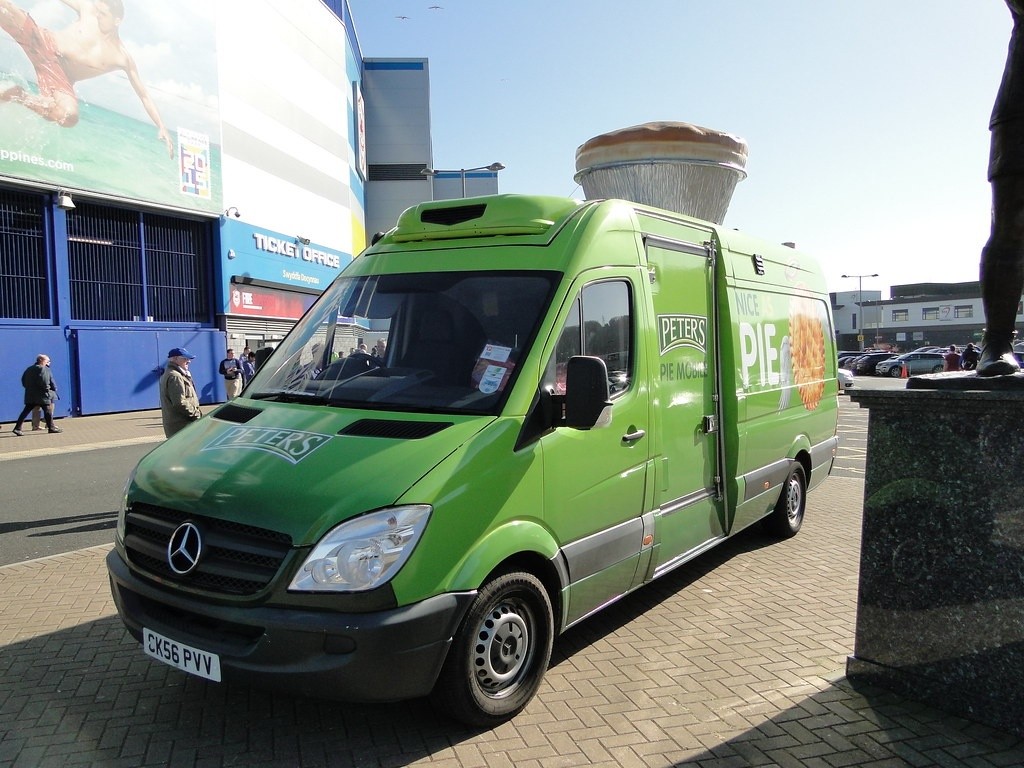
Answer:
[168,347,196,359]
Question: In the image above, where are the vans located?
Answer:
[106,194,840,729]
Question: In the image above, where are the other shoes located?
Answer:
[33,426,45,431]
[50,428,62,433]
[12,429,24,436]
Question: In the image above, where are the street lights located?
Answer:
[841,274,880,354]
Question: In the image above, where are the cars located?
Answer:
[876,356,902,375]
[838,346,983,370]
[880,352,946,378]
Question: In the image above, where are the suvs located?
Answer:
[854,353,900,376]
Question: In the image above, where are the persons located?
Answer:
[159,348,202,438]
[976,0,1024,376]
[330,337,387,363]
[238,346,255,389]
[959,342,979,371]
[219,349,245,400]
[12,354,63,436]
[0,0,175,160]
[303,342,325,380]
[943,345,962,372]
